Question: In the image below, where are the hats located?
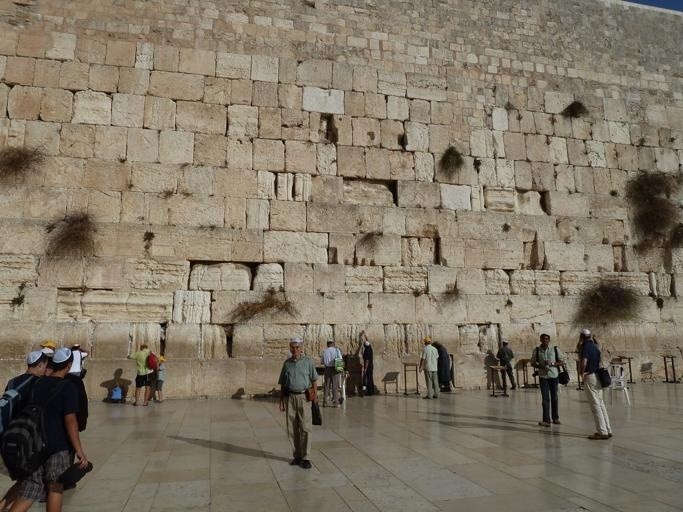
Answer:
[27,340,80,365]
[424,336,432,344]
[580,329,591,336]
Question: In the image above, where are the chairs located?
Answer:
[608,364,631,408]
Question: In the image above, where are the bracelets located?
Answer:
[551,362,554,366]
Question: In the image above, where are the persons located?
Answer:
[279,333,320,468]
[0,343,167,511]
[430,341,452,393]
[416,337,442,400]
[360,329,376,397]
[321,339,344,407]
[575,328,613,440]
[529,333,566,426]
[496,340,517,391]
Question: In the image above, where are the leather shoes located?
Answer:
[291,458,311,469]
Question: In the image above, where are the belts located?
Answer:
[290,391,305,394]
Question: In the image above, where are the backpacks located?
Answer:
[0,376,47,475]
[147,351,157,369]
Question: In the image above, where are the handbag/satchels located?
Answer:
[334,358,344,372]
[559,371,569,384]
[312,402,322,425]
[598,367,611,387]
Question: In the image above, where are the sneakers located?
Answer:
[539,419,560,427]
[588,433,612,440]
